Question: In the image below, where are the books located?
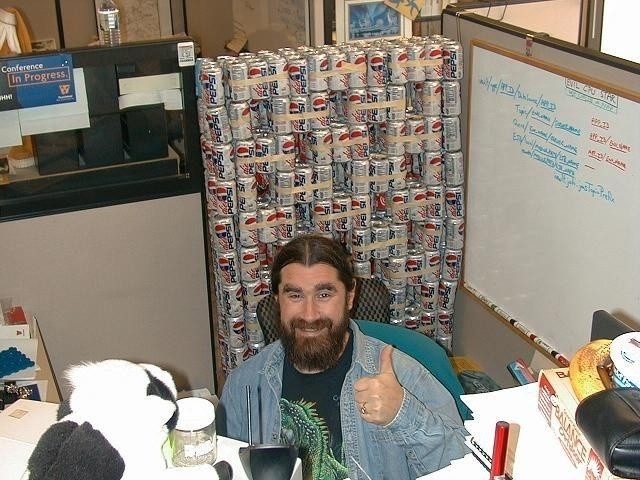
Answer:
[0,324,62,446]
[410,344,629,480]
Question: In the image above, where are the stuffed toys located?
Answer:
[22,353,180,480]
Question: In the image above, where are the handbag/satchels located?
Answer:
[575,388,640,479]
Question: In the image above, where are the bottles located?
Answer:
[97,0,121,48]
[167,396,217,469]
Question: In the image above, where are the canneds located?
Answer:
[194,34,465,379]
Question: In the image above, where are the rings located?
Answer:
[360,402,366,415]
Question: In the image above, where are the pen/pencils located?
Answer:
[460,280,570,367]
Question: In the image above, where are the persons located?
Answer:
[213,234,472,479]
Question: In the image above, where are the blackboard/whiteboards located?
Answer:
[459,38,640,367]
